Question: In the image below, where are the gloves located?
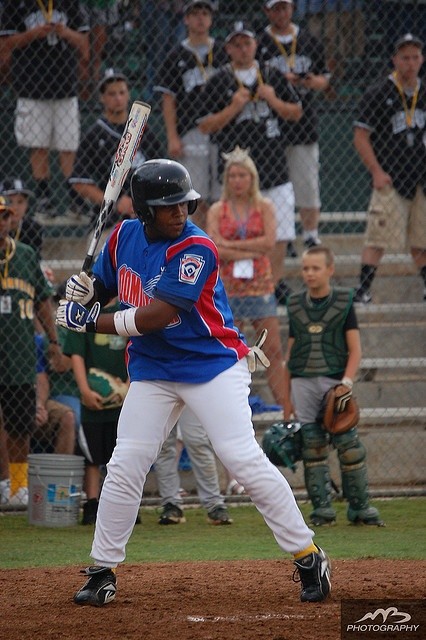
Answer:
[247,328,271,373]
[55,299,101,333]
[66,272,97,306]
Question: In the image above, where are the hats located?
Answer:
[266,0,295,10]
[96,67,130,90]
[393,33,425,52]
[0,175,36,199]
[0,194,16,215]
[182,1,214,14]
[225,19,256,43]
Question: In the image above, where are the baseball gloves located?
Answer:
[88,366,129,408]
[322,383,360,435]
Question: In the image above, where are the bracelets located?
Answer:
[340,376,356,391]
[114,311,129,340]
[124,306,144,339]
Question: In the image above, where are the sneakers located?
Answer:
[74,566,117,606]
[207,510,233,524]
[7,486,27,509]
[82,498,98,525]
[158,505,186,524]
[307,516,337,528]
[276,281,294,305]
[351,287,373,304]
[350,515,387,528]
[293,542,332,602]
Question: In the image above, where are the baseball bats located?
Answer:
[81,98,153,271]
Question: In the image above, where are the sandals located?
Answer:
[221,479,246,495]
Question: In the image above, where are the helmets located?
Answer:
[130,158,201,225]
[261,421,302,466]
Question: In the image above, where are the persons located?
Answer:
[3,178,42,257]
[90,3,127,74]
[53,158,331,604]
[155,406,232,525]
[68,75,170,260]
[351,32,425,301]
[205,145,293,407]
[284,247,381,527]
[255,0,330,256]
[191,19,302,305]
[155,1,231,228]
[2,333,75,509]
[61,299,143,525]
[0,196,61,512]
[0,0,92,213]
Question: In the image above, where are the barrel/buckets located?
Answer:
[26,450,85,530]
